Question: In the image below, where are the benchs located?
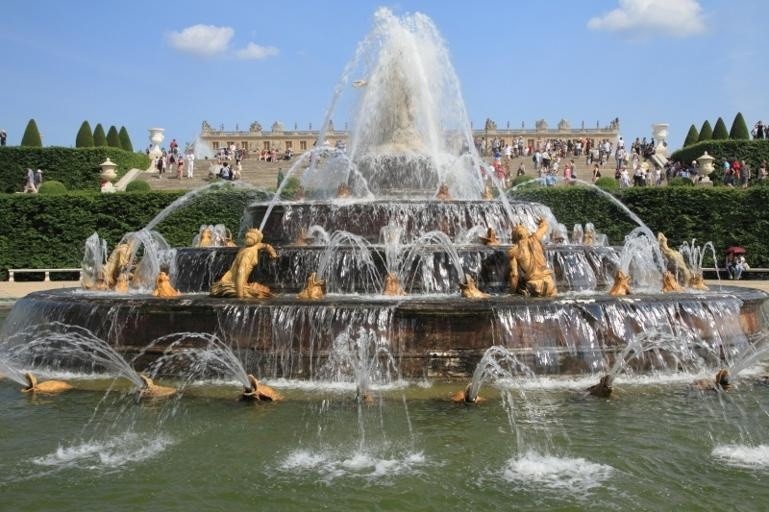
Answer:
[7,267,81,283]
[700,268,769,279]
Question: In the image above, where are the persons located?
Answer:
[23,168,41,193]
[511,215,555,298]
[474,121,769,188]
[105,232,136,286]
[210,227,280,299]
[726,252,745,279]
[0,129,7,145]
[146,139,348,181]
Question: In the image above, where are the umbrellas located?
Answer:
[725,247,746,253]
